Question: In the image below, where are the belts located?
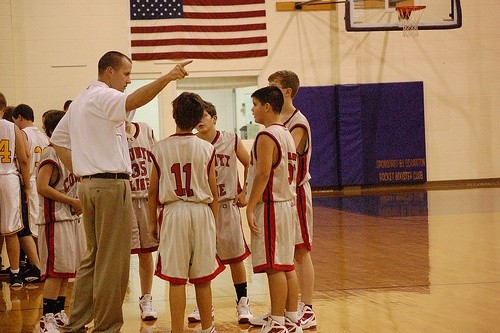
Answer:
[84,172,130,180]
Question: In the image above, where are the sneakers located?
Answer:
[0,265,12,275]
[236,297,253,323]
[55,309,89,332]
[297,301,317,329]
[248,308,272,326]
[283,312,303,333]
[9,267,24,287]
[22,262,41,282]
[187,302,215,321]
[140,293,157,320]
[261,316,289,333]
[39,313,61,333]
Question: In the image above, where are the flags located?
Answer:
[129,0,268,61]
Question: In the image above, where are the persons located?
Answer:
[246,86,302,333]
[147,92,218,333]
[188,102,253,324]
[0,92,74,287]
[249,70,318,330]
[37,111,87,333]
[50,51,193,333]
[125,122,160,320]
